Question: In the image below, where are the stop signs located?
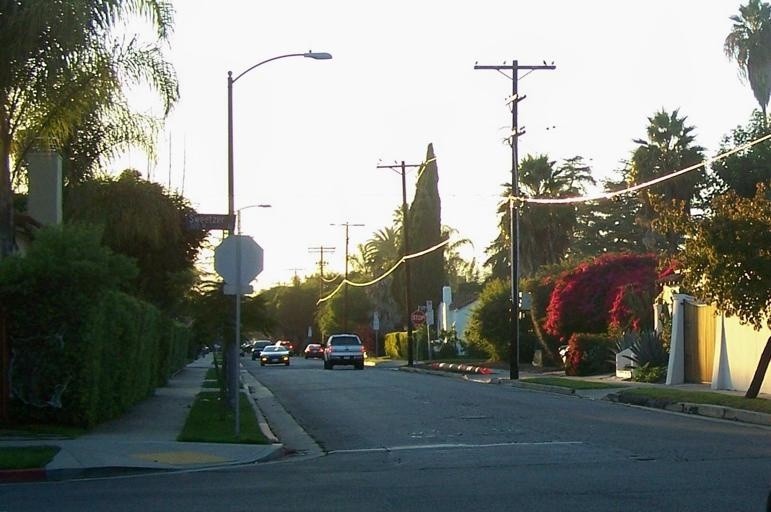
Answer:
[411,310,426,325]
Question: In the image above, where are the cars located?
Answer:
[275,340,294,357]
[305,343,324,359]
[260,345,290,366]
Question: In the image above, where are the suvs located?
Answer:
[251,340,272,360]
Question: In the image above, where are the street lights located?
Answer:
[236,204,271,234]
[228,50,332,435]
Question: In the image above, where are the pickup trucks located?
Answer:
[325,332,364,369]
